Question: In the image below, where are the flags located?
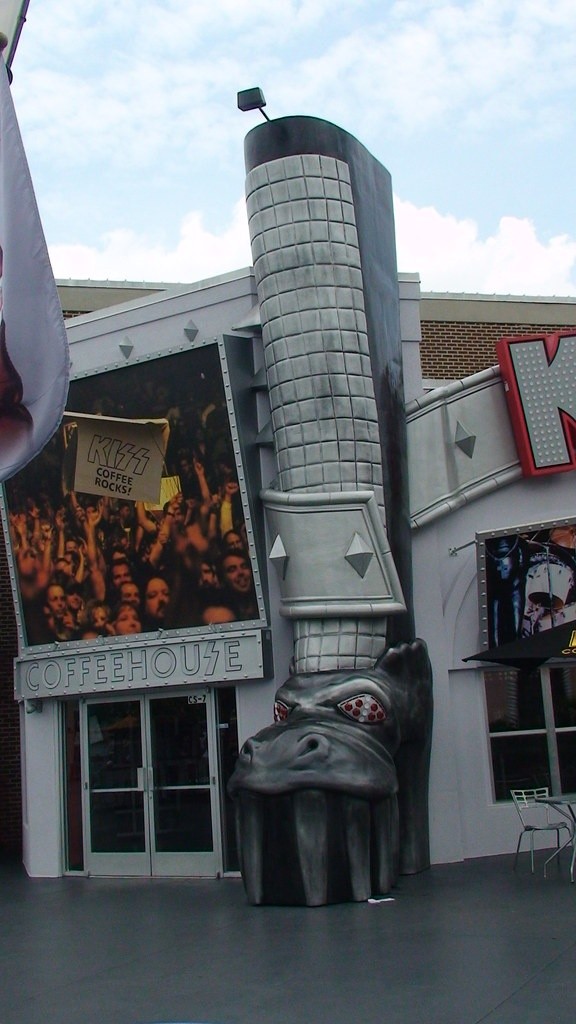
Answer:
[0,53,71,482]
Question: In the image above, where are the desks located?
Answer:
[535,796,576,884]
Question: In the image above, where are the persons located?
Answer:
[9,458,259,642]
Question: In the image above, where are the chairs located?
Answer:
[510,788,562,871]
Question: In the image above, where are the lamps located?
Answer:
[230,87,271,122]
